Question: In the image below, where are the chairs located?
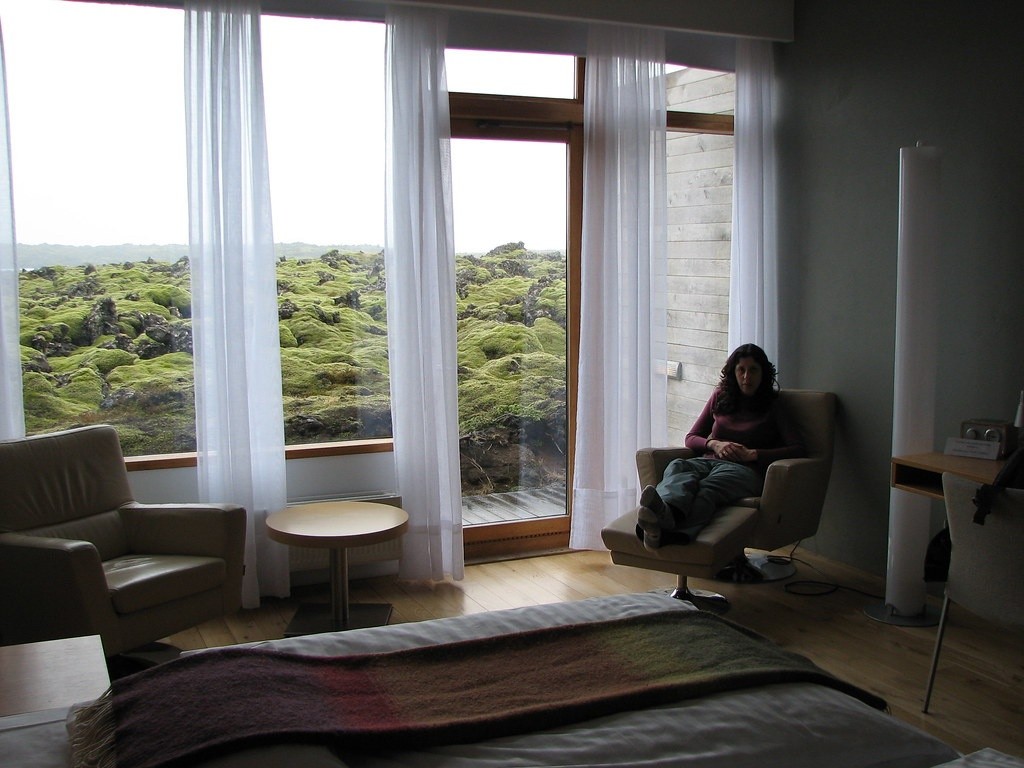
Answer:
[921,470,1024,716]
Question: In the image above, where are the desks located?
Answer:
[891,448,1023,530]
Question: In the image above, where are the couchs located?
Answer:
[601,389,842,616]
[1,423,247,681]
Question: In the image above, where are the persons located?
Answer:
[635,344,807,554]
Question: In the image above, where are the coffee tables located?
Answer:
[267,499,411,637]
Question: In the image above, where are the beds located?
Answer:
[66,591,969,767]
